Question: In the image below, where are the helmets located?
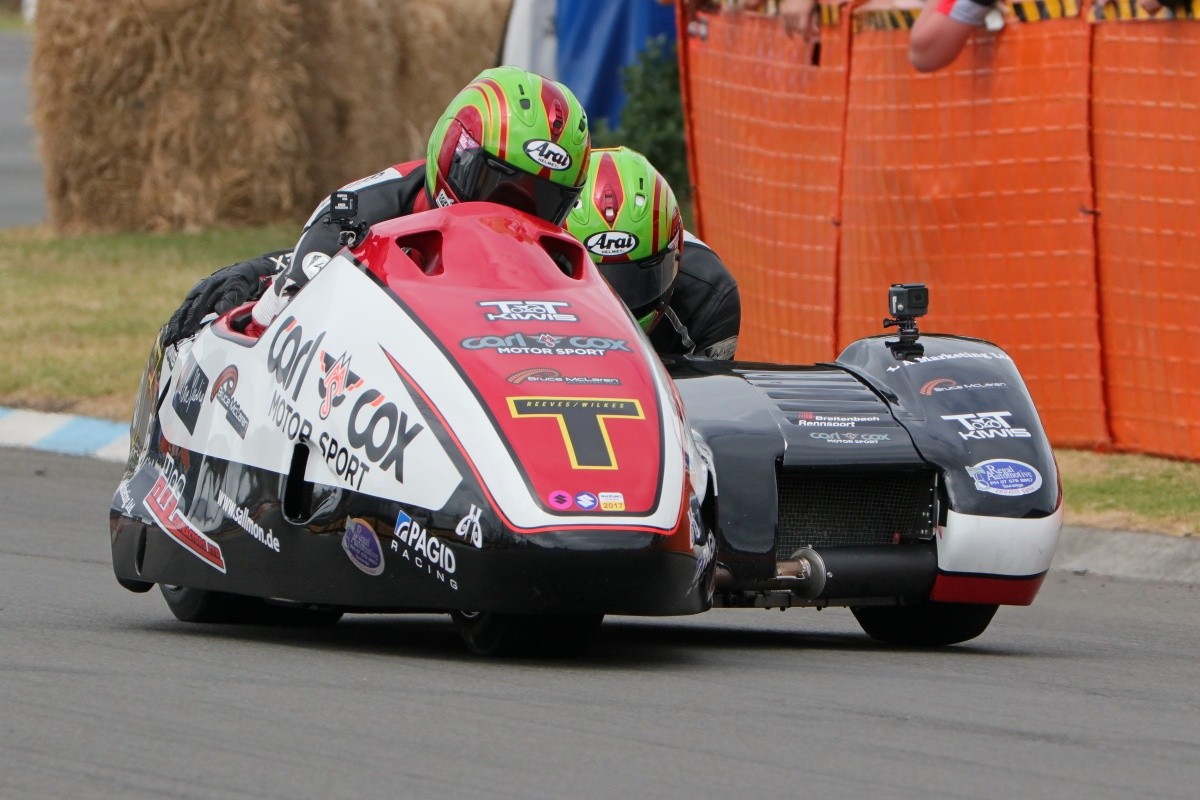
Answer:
[426,65,592,229]
[562,146,684,335]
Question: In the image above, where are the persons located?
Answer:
[723,0,1191,72]
[244,64,594,342]
[563,137,738,371]
